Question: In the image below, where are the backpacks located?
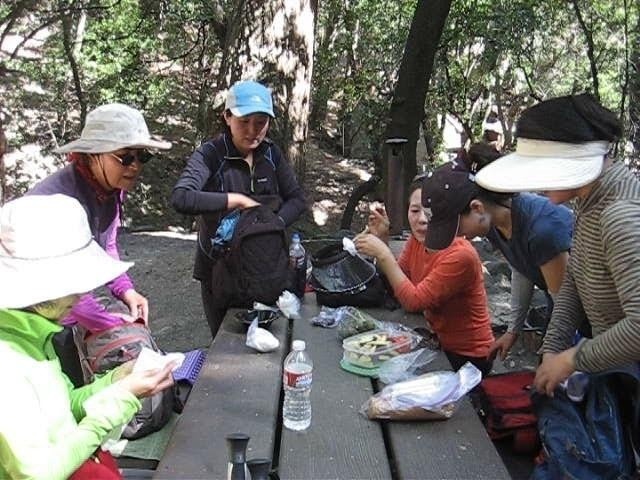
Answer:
[207,193,291,313]
[474,364,555,462]
[527,369,639,476]
[71,297,173,440]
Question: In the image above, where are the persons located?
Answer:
[355,174,498,377]
[474,95,640,456]
[172,82,307,341]
[421,143,578,362]
[24,101,150,333]
[0,194,175,480]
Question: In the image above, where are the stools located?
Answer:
[113,411,180,469]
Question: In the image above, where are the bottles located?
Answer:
[289,234,306,269]
[282,339,313,431]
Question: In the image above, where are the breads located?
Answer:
[368,395,454,421]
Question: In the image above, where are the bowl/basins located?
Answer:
[235,309,283,331]
[341,329,415,368]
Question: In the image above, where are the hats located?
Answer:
[52,103,172,155]
[475,138,612,192]
[222,80,275,118]
[0,193,135,310]
[421,162,475,250]
[309,242,376,292]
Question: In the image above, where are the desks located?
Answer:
[153,290,511,479]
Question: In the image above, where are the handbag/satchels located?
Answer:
[316,278,386,307]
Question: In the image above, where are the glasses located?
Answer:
[110,153,153,166]
